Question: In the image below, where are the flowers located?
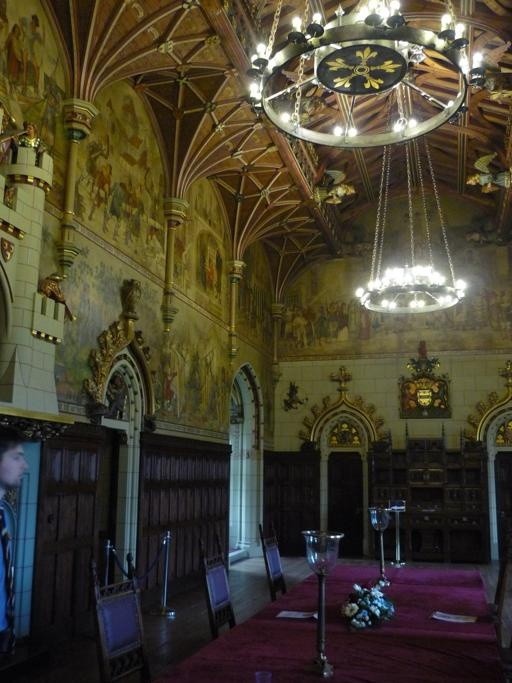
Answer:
[341,582,397,629]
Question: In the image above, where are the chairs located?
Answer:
[90,554,153,682]
[199,534,236,638]
[256,522,288,601]
[489,545,509,644]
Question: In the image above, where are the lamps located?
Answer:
[249,0,496,314]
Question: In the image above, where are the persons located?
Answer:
[0,421,30,655]
[11,119,47,167]
[87,162,114,225]
[282,286,511,349]
[150,369,163,415]
[355,591,373,627]
[162,366,177,402]
[103,181,129,242]
